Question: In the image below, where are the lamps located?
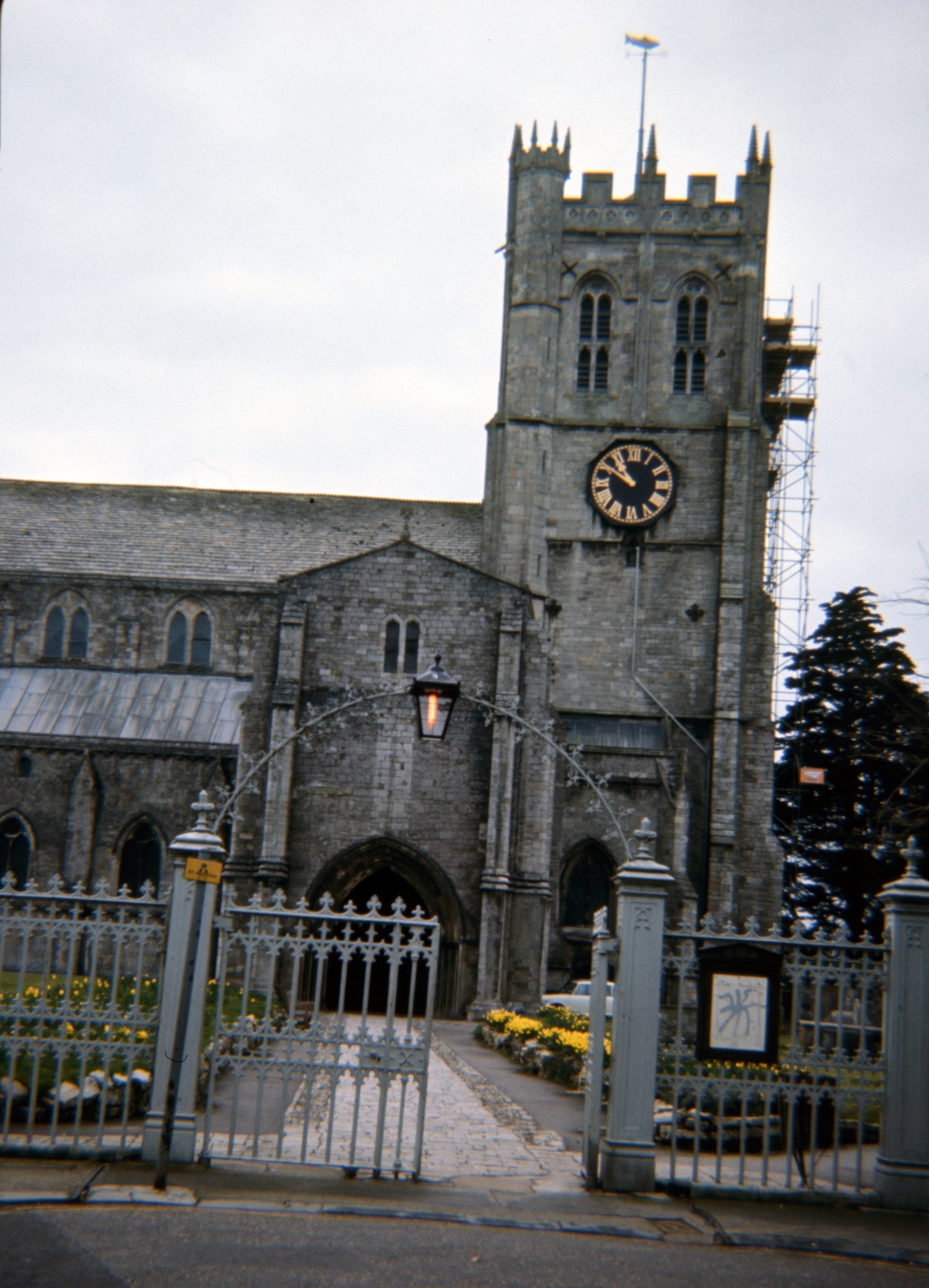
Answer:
[407,649,464,743]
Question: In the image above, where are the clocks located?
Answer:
[586,436,680,530]
